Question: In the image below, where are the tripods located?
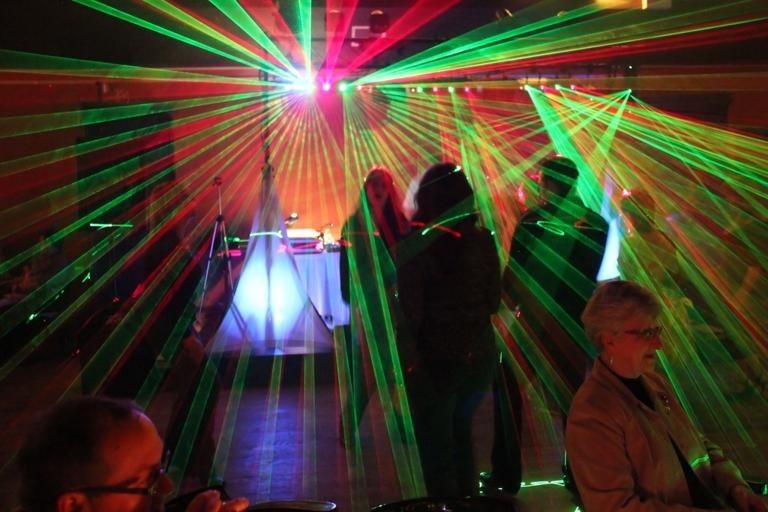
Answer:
[219,63,330,348]
[196,176,251,346]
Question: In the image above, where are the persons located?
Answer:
[487,155,609,501]
[339,163,415,450]
[21,393,252,511]
[618,175,768,397]
[2,179,226,466]
[394,162,501,502]
[564,281,768,512]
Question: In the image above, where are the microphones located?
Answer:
[284,214,298,228]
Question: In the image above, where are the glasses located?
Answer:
[625,326,663,337]
[86,450,171,498]
